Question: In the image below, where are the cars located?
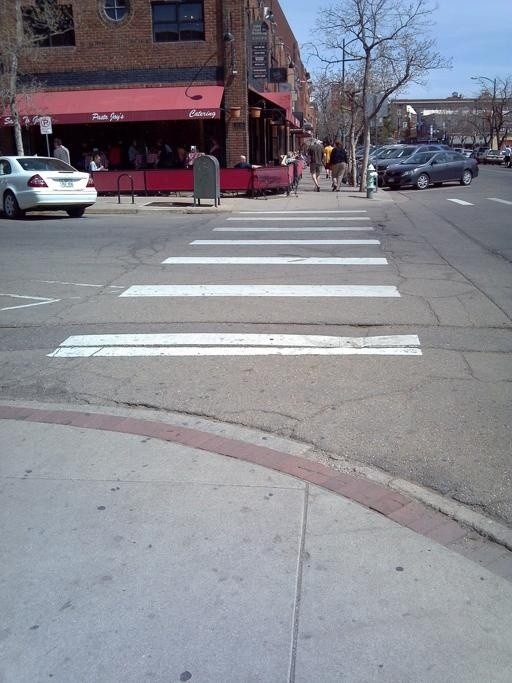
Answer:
[384,150,479,189]
[353,143,453,187]
[454,146,505,164]
[0,152,98,218]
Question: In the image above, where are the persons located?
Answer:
[234,155,260,168]
[308,139,347,191]
[210,138,221,154]
[54,139,70,165]
[177,142,188,160]
[109,138,173,169]
[503,141,512,167]
[77,141,109,171]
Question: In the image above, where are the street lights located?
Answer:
[341,33,381,83]
[469,75,497,104]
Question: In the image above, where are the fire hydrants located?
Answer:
[365,163,378,199]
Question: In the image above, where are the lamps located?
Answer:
[246,5,313,85]
[221,8,238,75]
